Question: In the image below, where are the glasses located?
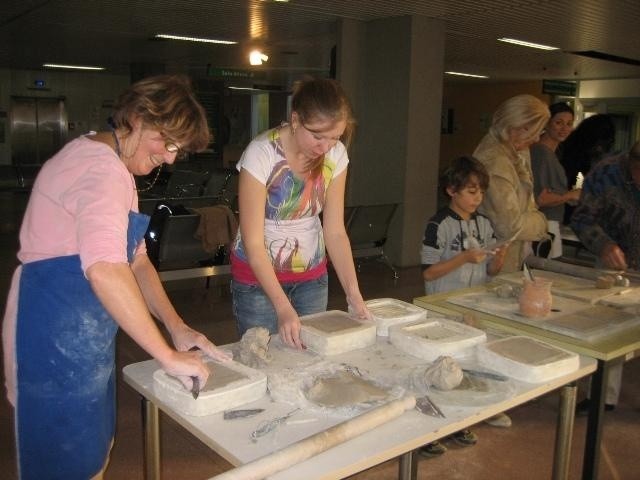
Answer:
[157,122,182,153]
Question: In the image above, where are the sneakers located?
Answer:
[419,439,447,458]
[448,429,478,446]
[485,412,512,427]
[575,397,616,416]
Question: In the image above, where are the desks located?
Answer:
[413,262,640,480]
[122,297,597,480]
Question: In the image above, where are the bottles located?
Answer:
[518,276,554,321]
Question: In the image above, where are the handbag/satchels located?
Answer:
[487,242,509,276]
[531,219,563,259]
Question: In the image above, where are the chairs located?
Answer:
[136,162,403,294]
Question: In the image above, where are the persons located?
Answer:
[555,111,617,230]
[0,67,236,479]
[467,91,556,432]
[566,138,640,420]
[525,100,583,264]
[226,74,378,352]
[408,153,513,461]
